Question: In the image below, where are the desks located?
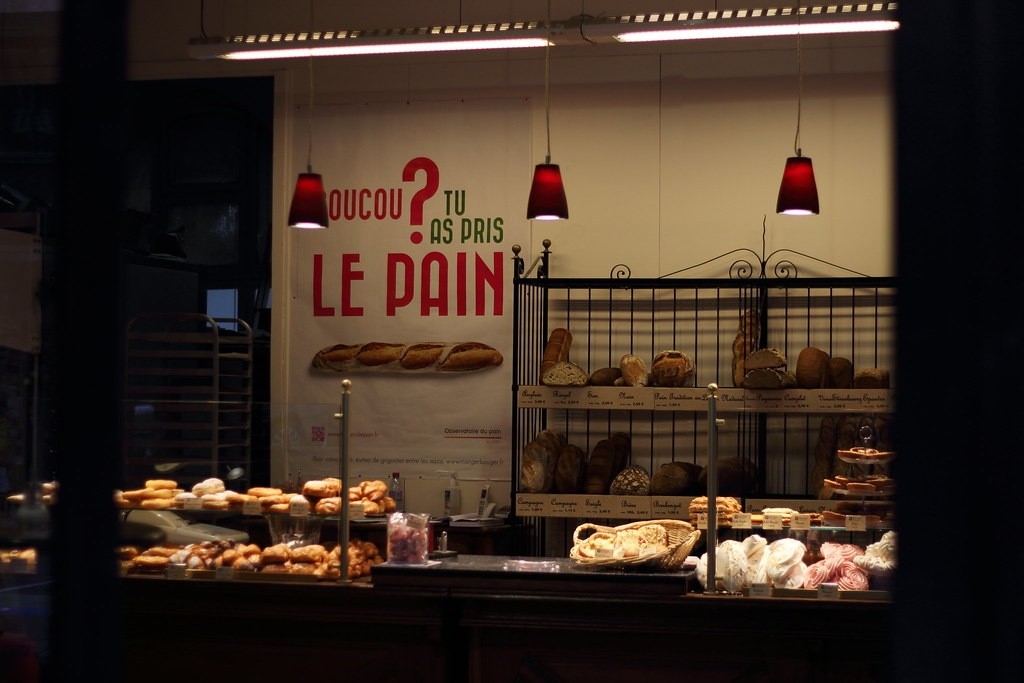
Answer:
[242,518,536,556]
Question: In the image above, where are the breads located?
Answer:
[0,478,395,580]
[578,524,668,560]
[310,341,503,375]
[732,309,888,390]
[688,495,741,527]
[821,447,891,493]
[517,327,763,497]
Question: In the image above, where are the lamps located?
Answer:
[777,0,819,216]
[187,0,900,61]
[288,1,328,229]
[526,1,569,222]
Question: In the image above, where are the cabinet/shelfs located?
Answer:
[510,238,898,562]
[125,312,253,494]
